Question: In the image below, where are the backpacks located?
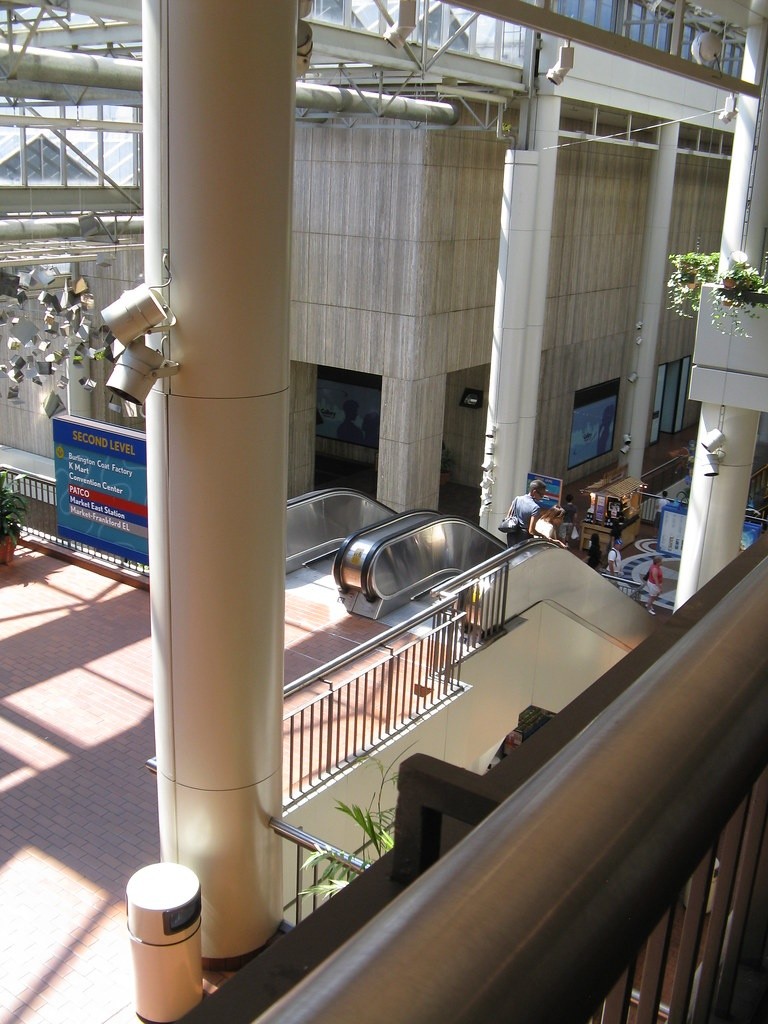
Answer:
[601,549,617,569]
[592,551,602,563]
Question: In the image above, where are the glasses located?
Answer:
[536,489,544,498]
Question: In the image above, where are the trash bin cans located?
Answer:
[125,864,204,1024]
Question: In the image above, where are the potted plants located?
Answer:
[0,470,36,564]
[667,252,768,338]
[439,448,455,485]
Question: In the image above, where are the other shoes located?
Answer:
[645,604,654,612]
[648,609,656,616]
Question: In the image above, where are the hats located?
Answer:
[614,539,623,545]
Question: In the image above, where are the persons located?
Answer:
[652,491,671,539]
[586,533,600,570]
[605,521,623,585]
[557,494,579,548]
[507,479,548,548]
[534,504,569,549]
[646,554,663,616]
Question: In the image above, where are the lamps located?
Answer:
[485,425,497,438]
[620,445,630,454]
[79,215,100,237]
[635,322,643,330]
[748,495,755,508]
[546,61,569,86]
[704,453,719,476]
[297,19,313,79]
[480,492,492,505]
[628,372,638,383]
[106,341,181,406]
[673,491,689,507]
[459,388,483,408]
[486,442,496,456]
[481,459,496,472]
[720,106,738,124]
[621,434,631,445]
[97,254,116,267]
[480,476,496,489]
[100,283,177,346]
[635,337,643,346]
[701,428,725,453]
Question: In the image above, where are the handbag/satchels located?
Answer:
[643,571,649,581]
[498,517,524,534]
[570,525,579,540]
